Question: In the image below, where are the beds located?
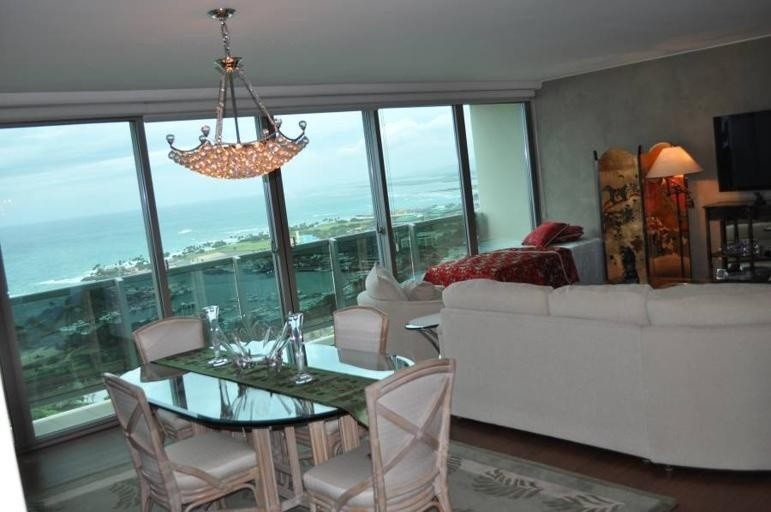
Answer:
[428,236,606,289]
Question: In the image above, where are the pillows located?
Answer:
[442,278,554,316]
[548,283,654,326]
[550,225,584,244]
[646,283,771,327]
[402,279,441,301]
[365,260,408,303]
[522,221,569,248]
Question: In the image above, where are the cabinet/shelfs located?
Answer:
[703,200,771,278]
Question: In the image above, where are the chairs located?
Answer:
[355,261,444,363]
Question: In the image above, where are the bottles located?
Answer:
[285,313,314,383]
[205,305,227,364]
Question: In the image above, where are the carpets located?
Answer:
[25,437,677,512]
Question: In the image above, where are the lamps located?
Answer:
[645,146,704,278]
[166,7,310,180]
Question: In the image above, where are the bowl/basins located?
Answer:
[217,327,291,372]
[225,384,304,427]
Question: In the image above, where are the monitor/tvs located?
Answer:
[713,110,771,192]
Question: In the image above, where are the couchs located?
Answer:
[438,277,771,471]
